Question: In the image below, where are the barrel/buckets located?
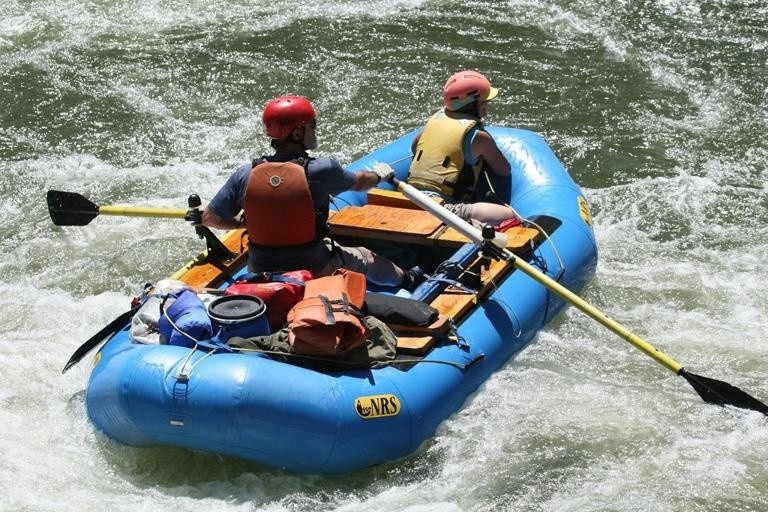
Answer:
[206,296,273,342]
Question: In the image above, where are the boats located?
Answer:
[86,118,598,477]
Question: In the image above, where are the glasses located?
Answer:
[307,118,318,131]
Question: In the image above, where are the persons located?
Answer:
[407,70,515,228]
[201,96,428,293]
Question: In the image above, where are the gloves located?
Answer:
[372,161,396,183]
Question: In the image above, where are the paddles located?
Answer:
[46,189,240,227]
[368,159,767,415]
[61,230,234,375]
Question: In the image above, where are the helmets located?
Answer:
[445,70,499,112]
[263,95,316,139]
